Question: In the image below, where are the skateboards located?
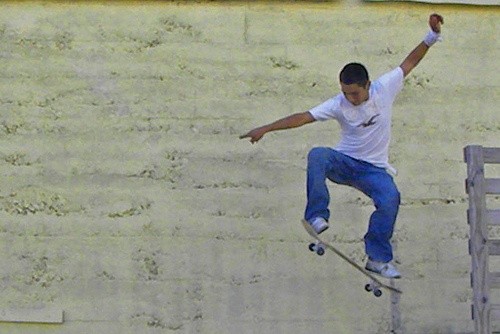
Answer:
[300,219,403,297]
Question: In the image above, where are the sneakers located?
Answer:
[307,216,329,235]
[364,256,402,279]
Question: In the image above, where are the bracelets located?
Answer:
[423,29,440,48]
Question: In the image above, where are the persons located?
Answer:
[239,13,443,279]
